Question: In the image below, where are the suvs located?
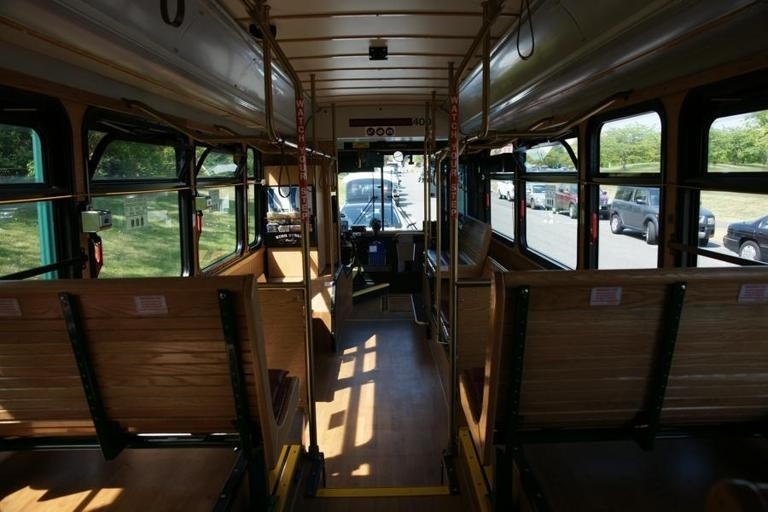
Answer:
[610,186,715,247]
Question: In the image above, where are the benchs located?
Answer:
[425,213,492,277]
[0,278,301,512]
[458,266,768,512]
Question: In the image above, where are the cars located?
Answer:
[497,179,610,220]
[340,161,403,229]
[723,215,768,263]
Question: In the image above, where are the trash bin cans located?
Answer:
[368,241,383,266]
